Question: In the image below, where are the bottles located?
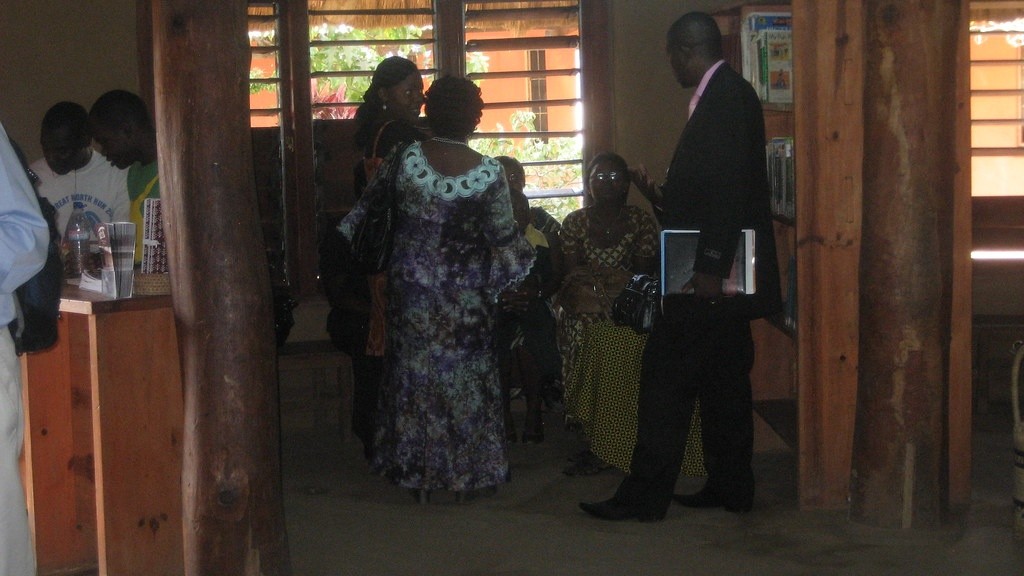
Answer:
[66,201,91,278]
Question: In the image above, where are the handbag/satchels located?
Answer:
[347,138,427,274]
[1,136,66,353]
[557,265,633,315]
[612,273,661,332]
[363,120,396,185]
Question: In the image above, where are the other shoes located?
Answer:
[504,419,516,443]
[522,408,540,443]
[545,382,563,402]
[579,477,666,520]
[671,472,753,512]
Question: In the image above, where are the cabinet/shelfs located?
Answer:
[750,102,798,464]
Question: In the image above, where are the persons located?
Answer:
[0,120,51,576]
[28,99,131,270]
[87,87,161,265]
[575,12,782,524]
[343,52,709,489]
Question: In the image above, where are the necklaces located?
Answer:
[592,208,621,234]
[431,136,467,148]
